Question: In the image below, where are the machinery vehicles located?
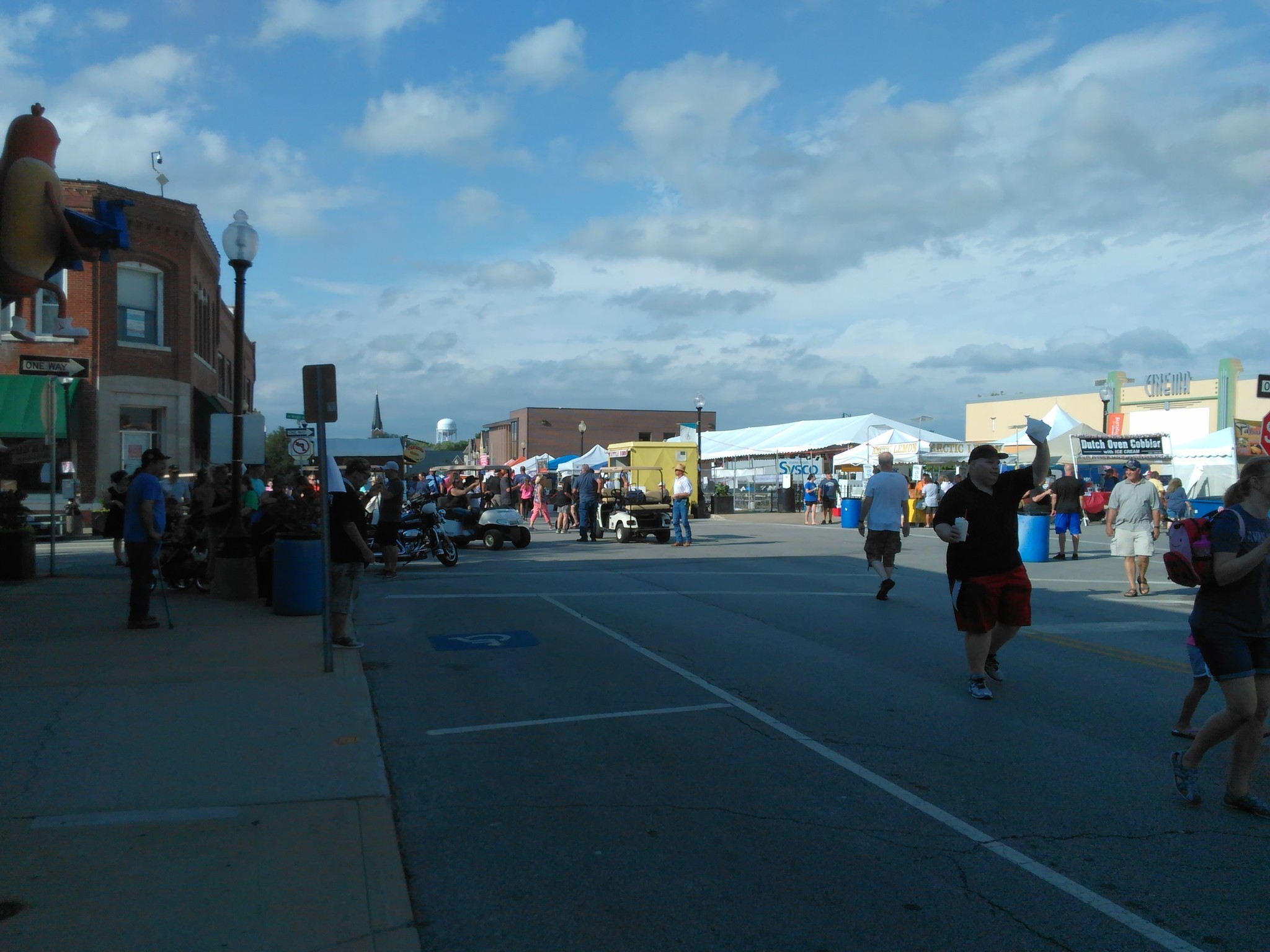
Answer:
[595,465,674,544]
[428,465,531,550]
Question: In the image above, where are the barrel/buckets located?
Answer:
[841,497,862,529]
[273,533,324,616]
[1018,511,1051,563]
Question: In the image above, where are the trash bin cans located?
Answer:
[272,535,325,618]
[1017,510,1050,563]
[840,497,862,528]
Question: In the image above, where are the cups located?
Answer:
[954,521,968,541]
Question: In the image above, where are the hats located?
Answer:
[658,482,665,487]
[604,474,609,479]
[1105,469,1114,473]
[672,464,688,474]
[379,461,399,471]
[1123,459,1142,470]
[560,471,573,480]
[169,465,182,473]
[140,448,171,460]
[1143,470,1152,476]
[969,444,1009,460]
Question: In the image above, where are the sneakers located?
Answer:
[374,568,388,576]
[1053,553,1066,560]
[1072,553,1078,560]
[577,537,588,542]
[820,520,826,524]
[331,636,366,647]
[985,653,1005,682]
[591,537,596,541]
[469,528,475,535]
[462,530,472,536]
[670,542,683,546]
[385,568,397,580]
[828,520,833,524]
[966,673,994,698]
[683,541,692,546]
[876,578,895,600]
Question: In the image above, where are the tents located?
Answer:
[661,413,963,513]
[486,444,609,495]
[829,427,1004,530]
[989,404,1170,465]
[1170,426,1239,502]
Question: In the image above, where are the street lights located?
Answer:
[578,420,587,457]
[59,376,73,461]
[221,207,261,498]
[1099,381,1112,434]
[693,390,710,519]
[521,441,526,458]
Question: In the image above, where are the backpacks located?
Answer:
[1161,508,1245,589]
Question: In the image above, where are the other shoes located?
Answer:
[520,509,583,534]
[128,617,160,629]
[115,559,127,566]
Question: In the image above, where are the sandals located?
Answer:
[1172,749,1202,804]
[1222,785,1270,818]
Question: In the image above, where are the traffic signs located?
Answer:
[286,427,315,438]
[19,354,90,380]
[285,414,305,420]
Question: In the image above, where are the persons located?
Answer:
[671,462,693,547]
[1106,459,1161,598]
[1171,634,1214,741]
[932,419,1051,699]
[1050,463,1085,560]
[373,460,405,578]
[104,469,671,596]
[329,455,375,648]
[817,470,841,524]
[1102,469,1120,492]
[1142,470,1152,480]
[1146,471,1166,529]
[1164,477,1193,536]
[122,448,171,629]
[857,451,910,601]
[873,468,964,528]
[804,473,819,525]
[1017,467,1055,515]
[1170,453,1270,820]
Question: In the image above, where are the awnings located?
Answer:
[195,388,229,413]
[0,372,67,440]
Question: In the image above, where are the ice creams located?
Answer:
[955,517,969,542]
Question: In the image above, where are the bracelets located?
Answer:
[858,519,864,523]
[1189,505,1191,508]
[1154,526,1160,529]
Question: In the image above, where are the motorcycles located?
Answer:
[364,491,458,567]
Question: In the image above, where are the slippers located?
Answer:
[1137,576,1150,594]
[1172,725,1201,739]
[1124,588,1138,597]
[924,525,931,528]
